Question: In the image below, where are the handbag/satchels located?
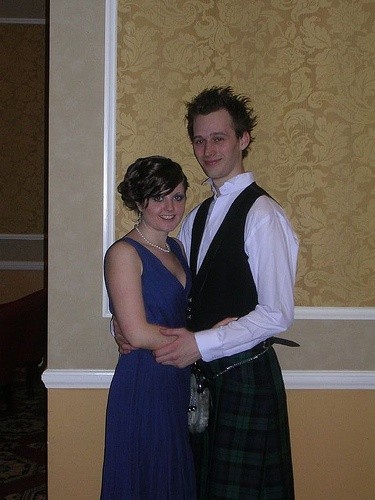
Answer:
[186,360,211,435]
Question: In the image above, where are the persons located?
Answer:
[110,85,299,500]
[100,156,236,500]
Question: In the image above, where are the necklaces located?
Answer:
[134,224,171,253]
